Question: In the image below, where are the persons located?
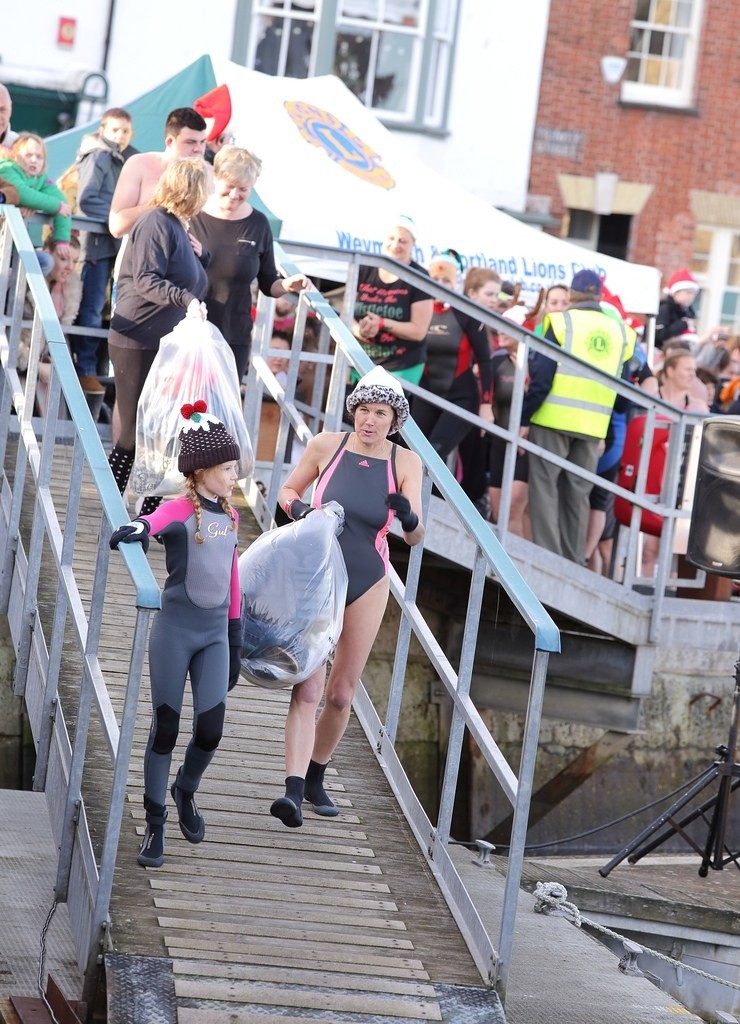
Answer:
[0,84,740,866]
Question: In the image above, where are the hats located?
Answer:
[501,305,529,324]
[347,366,410,436]
[670,327,698,342]
[178,400,241,471]
[390,213,416,242]
[570,268,603,294]
[430,248,463,274]
[663,268,699,295]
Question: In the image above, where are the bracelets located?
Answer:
[379,316,384,329]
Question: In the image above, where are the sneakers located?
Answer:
[304,760,338,817]
[136,794,169,867]
[171,766,206,843]
[270,774,304,829]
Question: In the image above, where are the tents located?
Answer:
[32,54,660,576]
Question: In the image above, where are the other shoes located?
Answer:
[78,374,106,394]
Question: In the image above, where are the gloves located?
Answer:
[385,492,418,532]
[290,499,315,520]
[108,517,150,554]
[228,617,241,693]
